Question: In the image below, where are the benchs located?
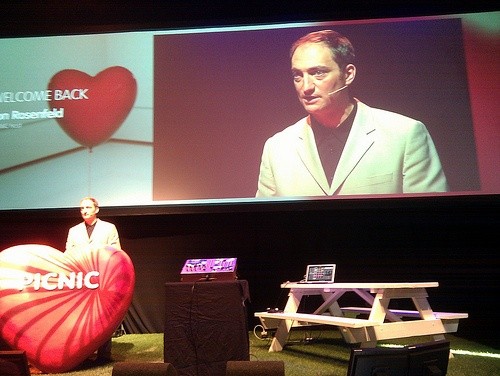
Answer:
[254,307,468,330]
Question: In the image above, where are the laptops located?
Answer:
[297,263,336,284]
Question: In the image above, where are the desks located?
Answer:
[268,282,468,358]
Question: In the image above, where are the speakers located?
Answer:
[112,361,178,376]
[226,361,285,376]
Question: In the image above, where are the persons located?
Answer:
[65,197,121,366]
[255,30,451,197]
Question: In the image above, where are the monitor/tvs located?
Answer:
[347,348,408,376]
[404,340,450,376]
